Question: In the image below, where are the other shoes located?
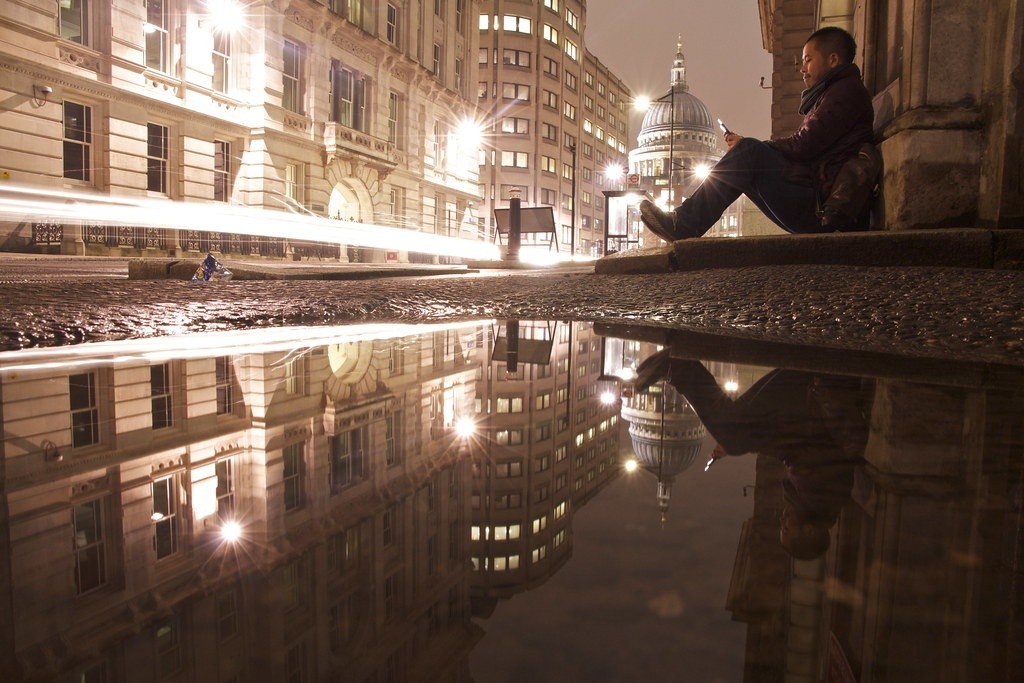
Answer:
[640,200,699,242]
[633,348,694,392]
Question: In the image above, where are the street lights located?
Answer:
[624,381,666,485]
[635,85,675,215]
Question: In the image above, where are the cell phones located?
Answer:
[703,457,715,471]
[717,118,732,135]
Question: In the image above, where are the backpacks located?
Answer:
[814,143,881,232]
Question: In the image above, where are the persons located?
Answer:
[640,27,874,242]
[635,349,877,560]
